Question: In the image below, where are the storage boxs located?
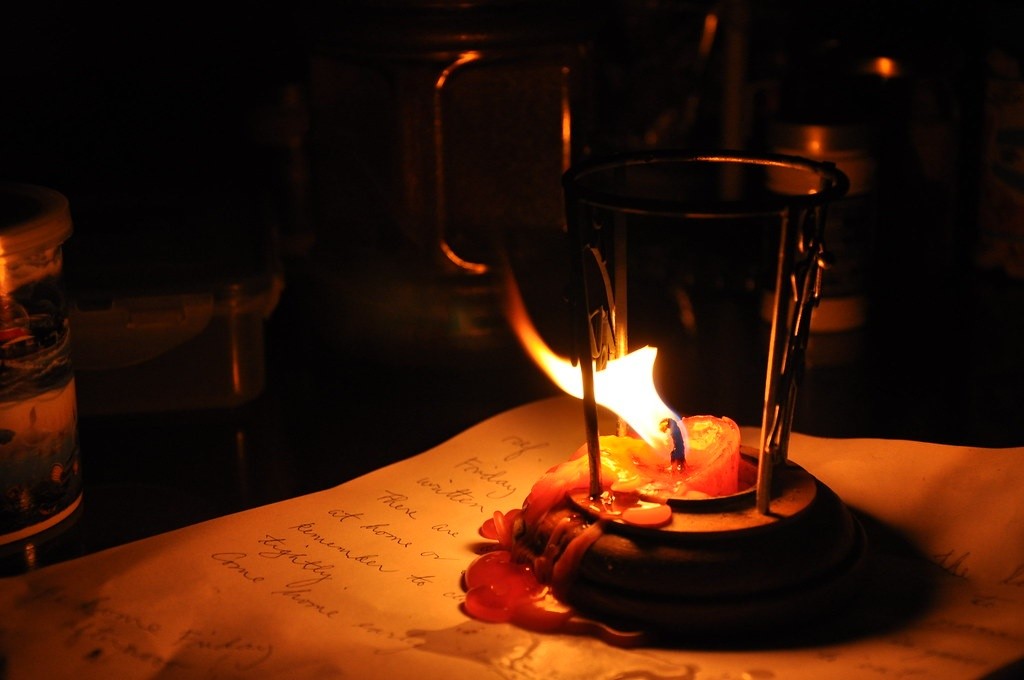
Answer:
[72,258,286,421]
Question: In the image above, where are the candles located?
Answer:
[633,416,741,497]
